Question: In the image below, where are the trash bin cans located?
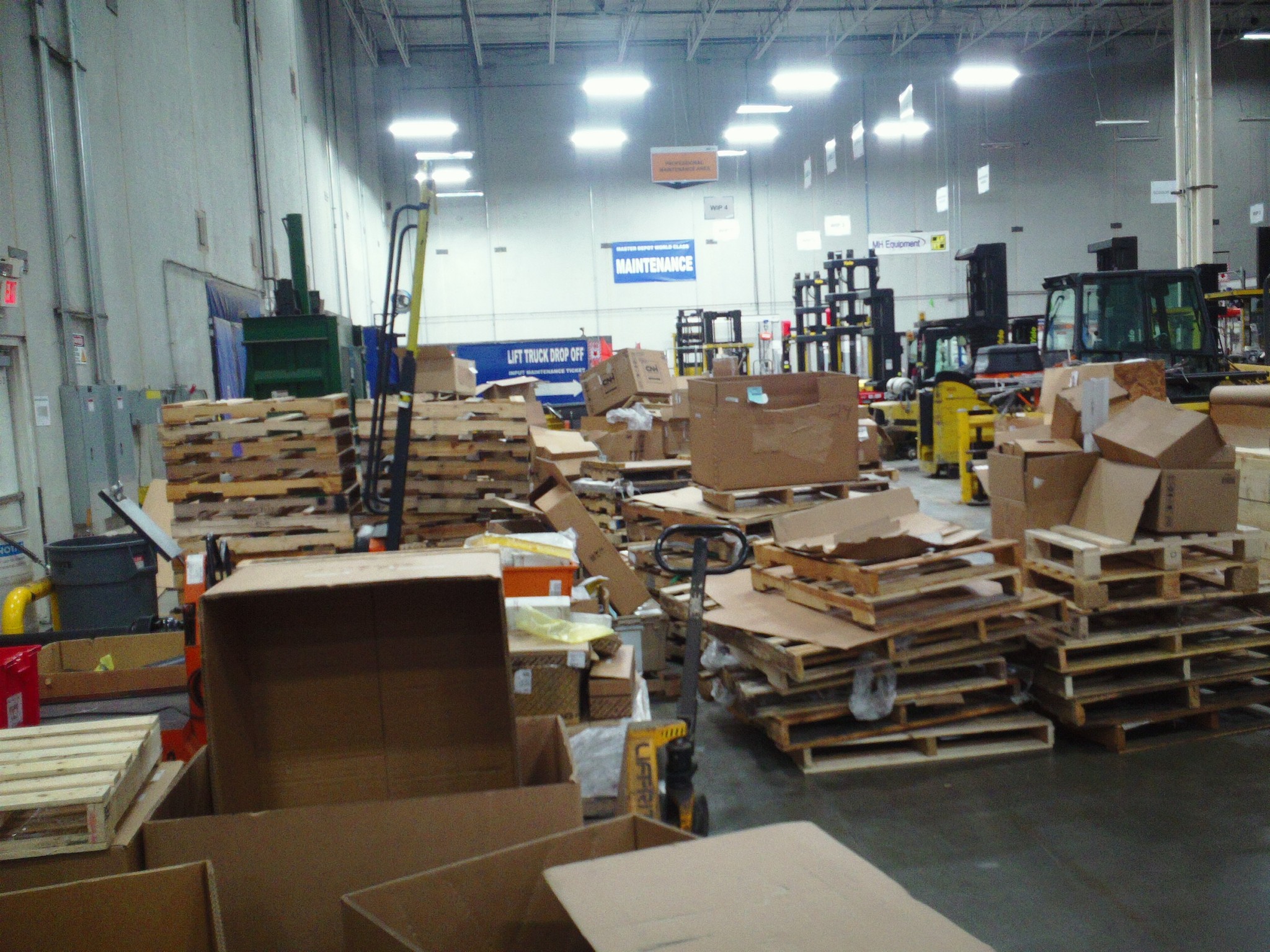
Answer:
[44,533,161,629]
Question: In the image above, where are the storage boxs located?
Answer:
[1,340,1246,952]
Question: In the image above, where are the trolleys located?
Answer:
[582,525,751,835]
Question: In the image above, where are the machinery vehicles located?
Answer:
[671,251,1260,509]
